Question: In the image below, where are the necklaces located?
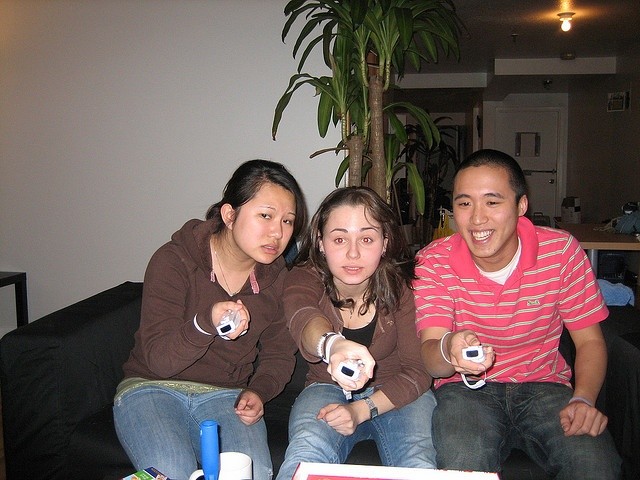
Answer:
[340,291,364,320]
[214,235,242,295]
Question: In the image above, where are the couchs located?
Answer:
[0,280,640,480]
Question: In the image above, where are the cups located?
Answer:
[190,452,252,480]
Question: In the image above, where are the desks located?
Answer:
[0,270,28,329]
[556,222,640,277]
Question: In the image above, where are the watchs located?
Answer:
[362,397,378,420]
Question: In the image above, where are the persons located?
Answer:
[113,160,308,480]
[275,186,438,480]
[411,149,621,480]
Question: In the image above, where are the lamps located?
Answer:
[557,12,575,32]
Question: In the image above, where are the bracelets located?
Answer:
[317,330,336,363]
[568,397,593,407]
